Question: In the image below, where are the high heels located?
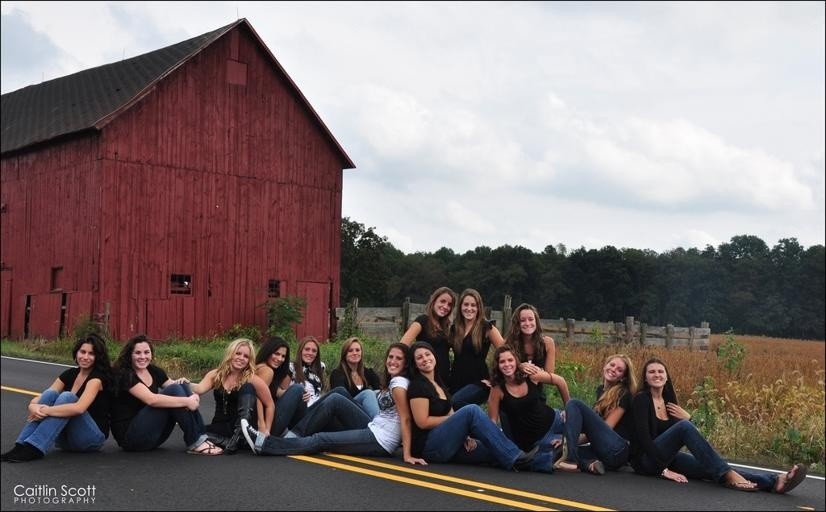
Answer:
[512,446,539,473]
[552,437,566,471]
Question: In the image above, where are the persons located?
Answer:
[399,286,456,396]
[330,335,381,399]
[173,338,276,456]
[241,341,428,465]
[288,335,328,417]
[1,333,115,462]
[447,289,506,413]
[109,334,225,456]
[408,341,554,473]
[490,301,806,494]
[237,335,307,450]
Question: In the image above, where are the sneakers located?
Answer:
[589,460,604,475]
[241,419,258,454]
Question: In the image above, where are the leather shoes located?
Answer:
[1,445,40,462]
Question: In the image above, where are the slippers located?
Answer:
[780,465,806,494]
[186,441,222,456]
[725,481,760,492]
